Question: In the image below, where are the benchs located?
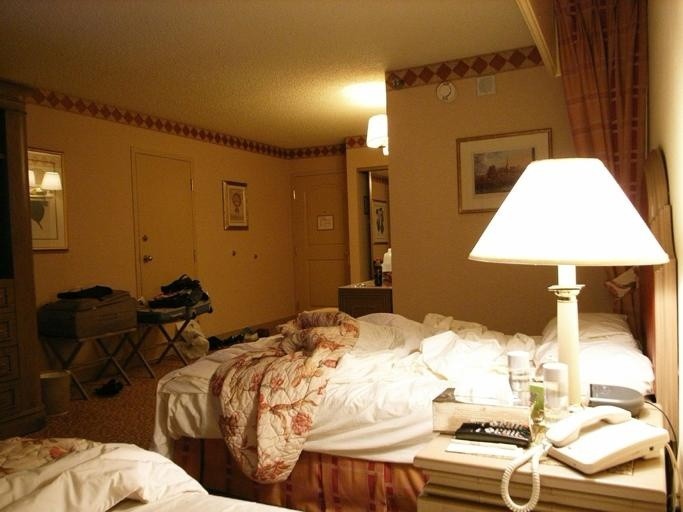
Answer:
[45,313,198,400]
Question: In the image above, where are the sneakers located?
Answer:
[207,327,269,350]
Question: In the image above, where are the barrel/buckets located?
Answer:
[382,248,392,286]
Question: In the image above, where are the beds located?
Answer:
[152,149,678,511]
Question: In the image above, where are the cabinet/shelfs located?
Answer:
[0,88,47,442]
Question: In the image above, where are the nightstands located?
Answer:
[411,402,667,512]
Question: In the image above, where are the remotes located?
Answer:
[466,420,531,436]
[455,421,531,448]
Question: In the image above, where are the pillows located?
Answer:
[536,309,653,397]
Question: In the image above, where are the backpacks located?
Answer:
[148,274,203,309]
[176,320,210,360]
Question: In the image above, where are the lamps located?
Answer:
[465,157,670,406]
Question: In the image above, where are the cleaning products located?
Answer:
[375,258,382,286]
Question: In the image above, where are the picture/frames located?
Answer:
[220,179,248,231]
[457,127,554,214]
[371,199,389,246]
[26,147,69,253]
[315,214,334,231]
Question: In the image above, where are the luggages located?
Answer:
[36,290,138,338]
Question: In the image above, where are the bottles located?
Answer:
[507,350,534,407]
[542,362,568,430]
[382,248,392,272]
[375,266,382,286]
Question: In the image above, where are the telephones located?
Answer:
[545,405,669,475]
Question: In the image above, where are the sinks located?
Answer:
[343,280,391,287]
